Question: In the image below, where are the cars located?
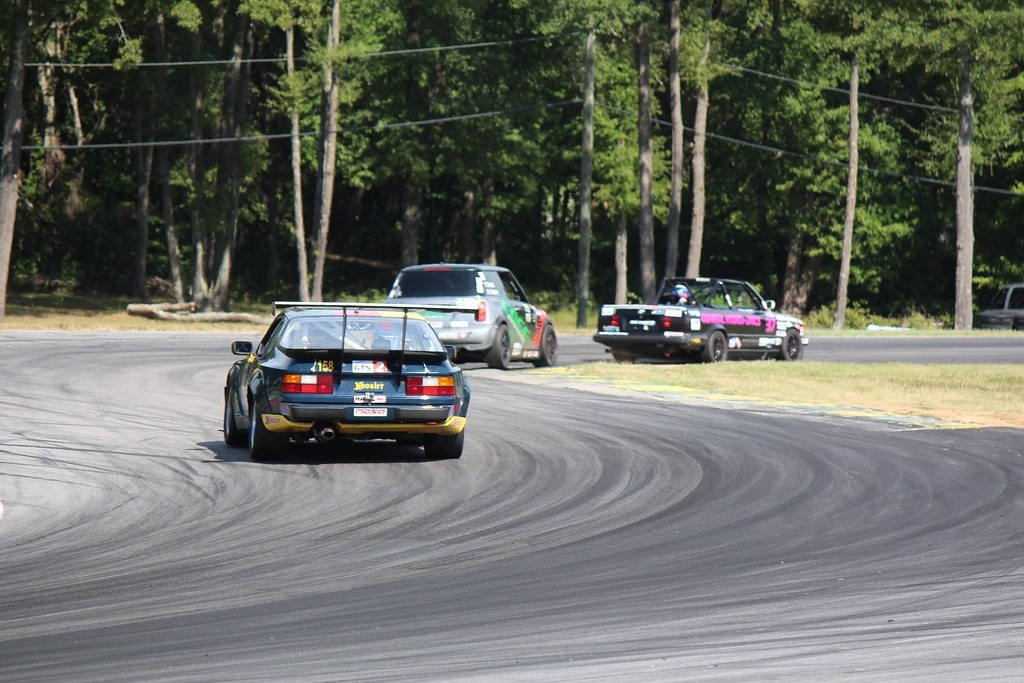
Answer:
[223,304,471,464]
[387,263,559,370]
[595,276,803,366]
[975,285,1024,328]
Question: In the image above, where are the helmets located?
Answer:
[672,285,689,299]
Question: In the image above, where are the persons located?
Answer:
[665,284,702,307]
